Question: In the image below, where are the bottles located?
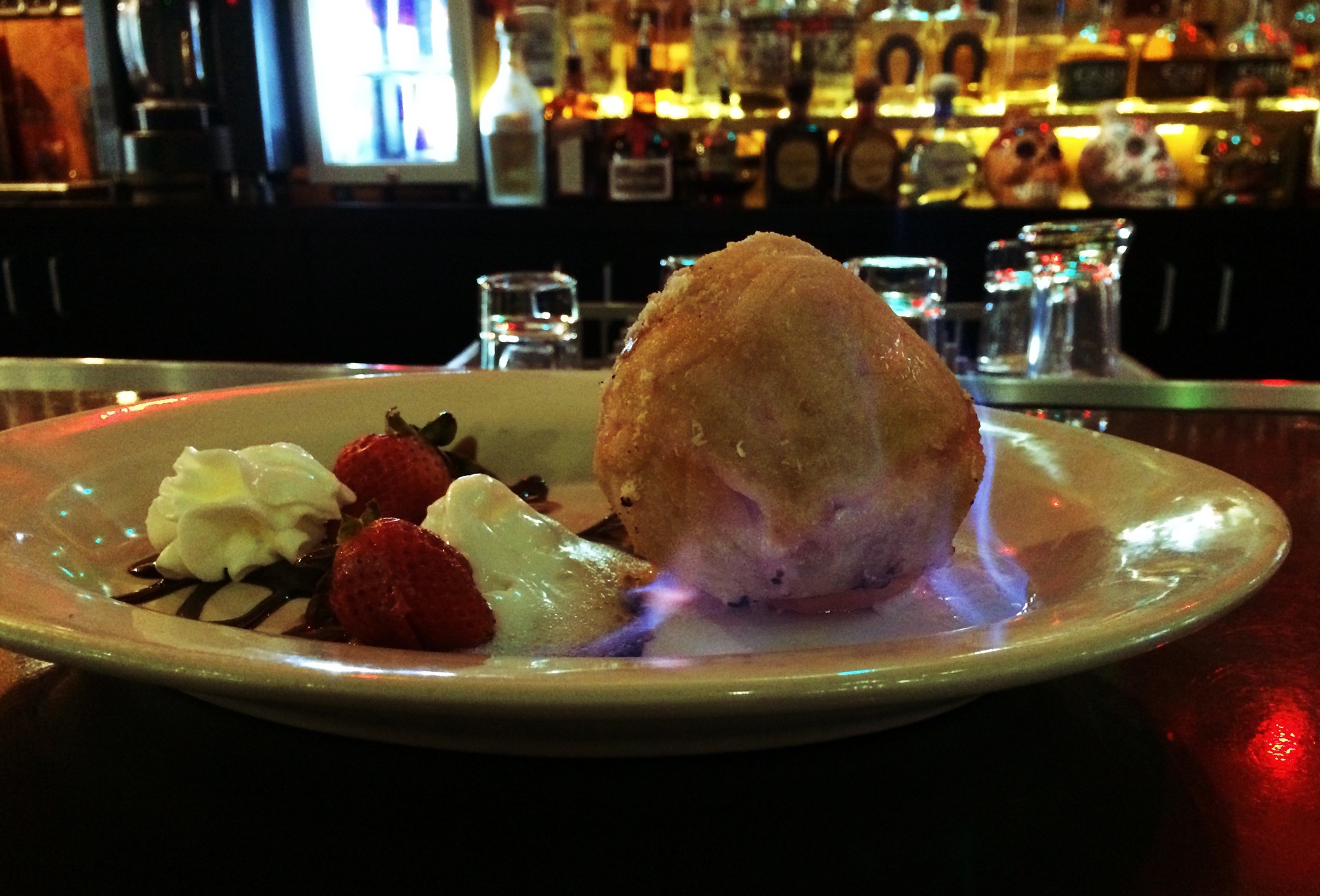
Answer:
[932,0,1001,115]
[858,0,938,118]
[765,74,833,208]
[1205,82,1289,206]
[480,18,544,207]
[564,0,617,93]
[735,0,802,98]
[682,84,760,206]
[512,0,557,87]
[1076,114,1171,207]
[1055,0,1296,105]
[606,47,674,204]
[835,80,900,212]
[805,0,858,102]
[546,56,601,206]
[983,74,1066,207]
[623,0,670,93]
[900,73,980,210]
[126,101,240,204]
[685,0,734,96]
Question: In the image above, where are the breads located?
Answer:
[591,229,988,617]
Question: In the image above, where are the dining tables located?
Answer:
[0,359,1320,896]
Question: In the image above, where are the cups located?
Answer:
[478,274,579,370]
[1018,220,1135,379]
[975,234,1080,372]
[847,257,946,359]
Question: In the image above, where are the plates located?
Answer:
[0,370,1291,756]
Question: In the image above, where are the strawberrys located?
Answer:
[292,500,499,652]
[331,408,458,524]
